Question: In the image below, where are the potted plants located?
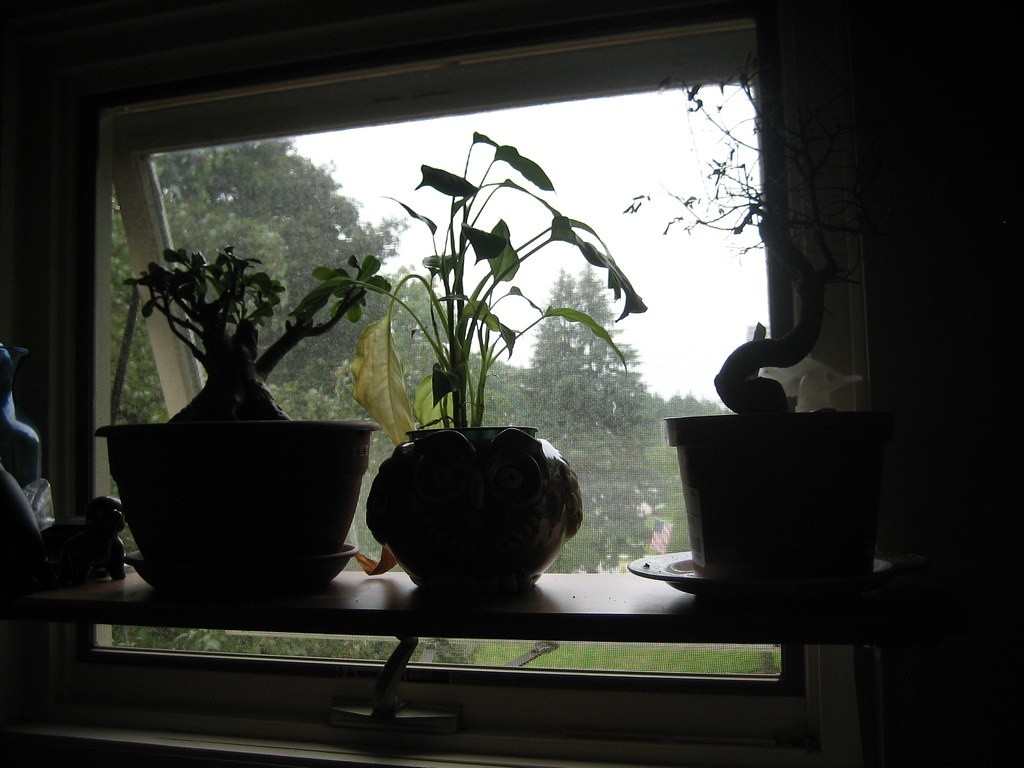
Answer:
[285,132,645,601]
[95,245,393,608]
[611,54,936,605]
